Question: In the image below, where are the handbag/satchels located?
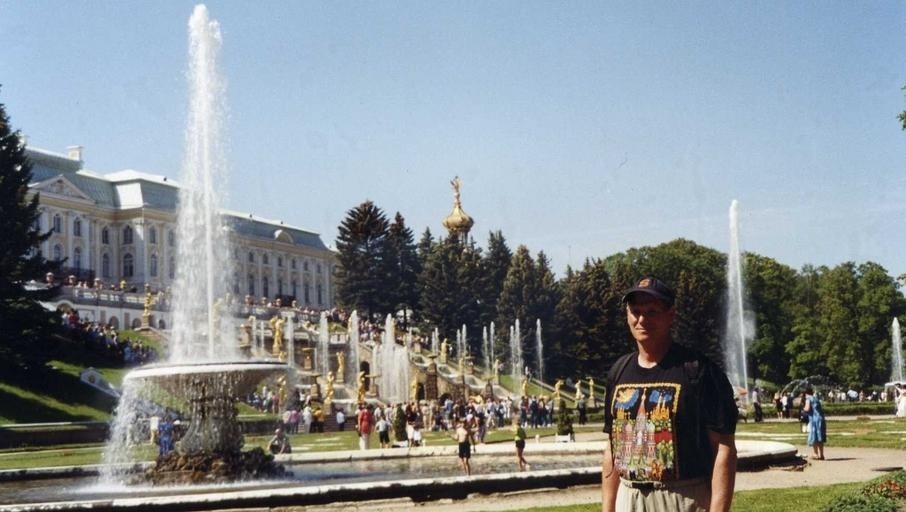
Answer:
[798,410,811,425]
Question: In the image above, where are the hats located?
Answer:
[621,275,676,308]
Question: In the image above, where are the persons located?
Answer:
[733,383,890,425]
[228,302,588,477]
[802,390,827,460]
[55,306,157,367]
[76,280,137,293]
[124,405,182,456]
[600,276,739,512]
